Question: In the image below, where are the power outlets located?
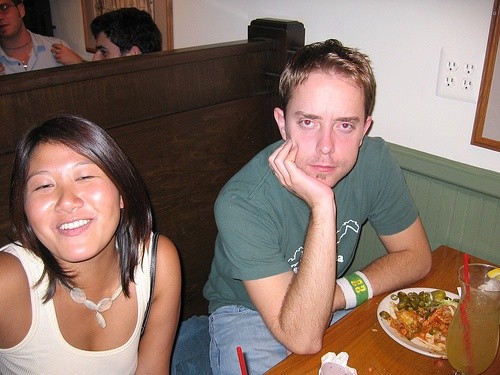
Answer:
[436,47,484,104]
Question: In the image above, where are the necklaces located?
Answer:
[57,277,125,328]
[4,39,32,63]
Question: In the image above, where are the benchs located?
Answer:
[0,18,305,321]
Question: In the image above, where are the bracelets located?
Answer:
[335,270,373,310]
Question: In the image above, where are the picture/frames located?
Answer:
[80,0,173,55]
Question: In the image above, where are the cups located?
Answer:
[444,263,500,375]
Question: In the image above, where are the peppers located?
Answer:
[379,291,431,319]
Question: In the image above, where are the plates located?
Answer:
[376,287,460,360]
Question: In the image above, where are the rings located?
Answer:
[55,48,56,53]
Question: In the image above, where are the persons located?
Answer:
[0,113,182,375]
[90,7,162,62]
[0,0,85,75]
[203,39,433,375]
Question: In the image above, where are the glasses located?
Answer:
[0,3,18,11]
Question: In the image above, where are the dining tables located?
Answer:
[263,244,500,375]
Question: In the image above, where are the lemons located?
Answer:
[487,268,500,282]
